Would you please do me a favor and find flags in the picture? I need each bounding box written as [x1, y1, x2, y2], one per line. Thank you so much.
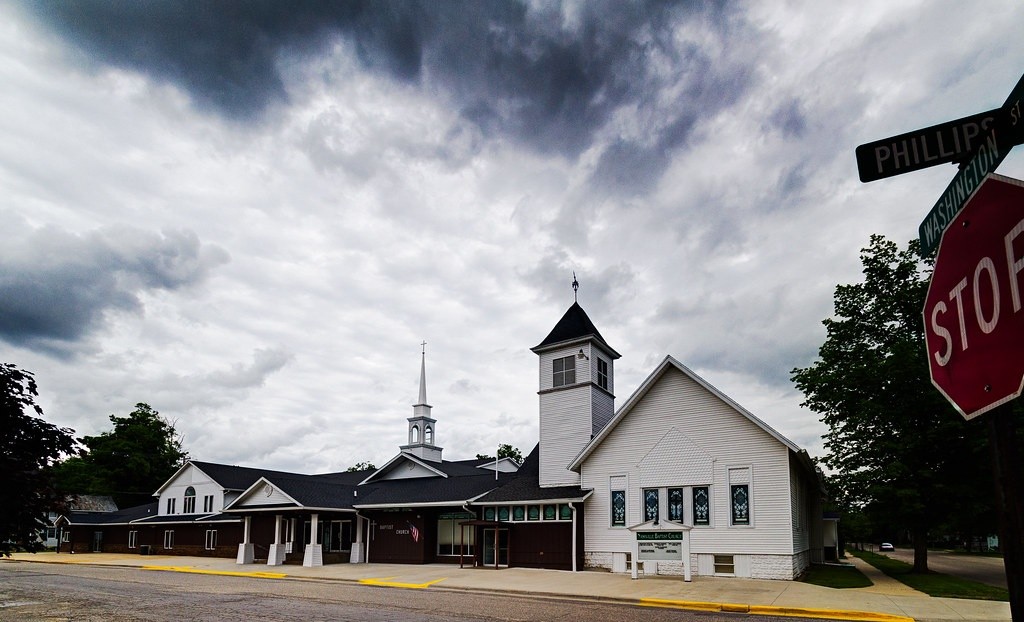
[409, 523, 419, 543]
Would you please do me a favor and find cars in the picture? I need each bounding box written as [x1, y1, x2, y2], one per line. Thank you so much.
[879, 543, 894, 551]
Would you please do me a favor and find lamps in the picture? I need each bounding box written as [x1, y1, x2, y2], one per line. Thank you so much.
[577, 349, 589, 360]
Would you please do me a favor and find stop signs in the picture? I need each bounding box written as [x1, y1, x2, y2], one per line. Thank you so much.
[921, 173, 1024, 421]
